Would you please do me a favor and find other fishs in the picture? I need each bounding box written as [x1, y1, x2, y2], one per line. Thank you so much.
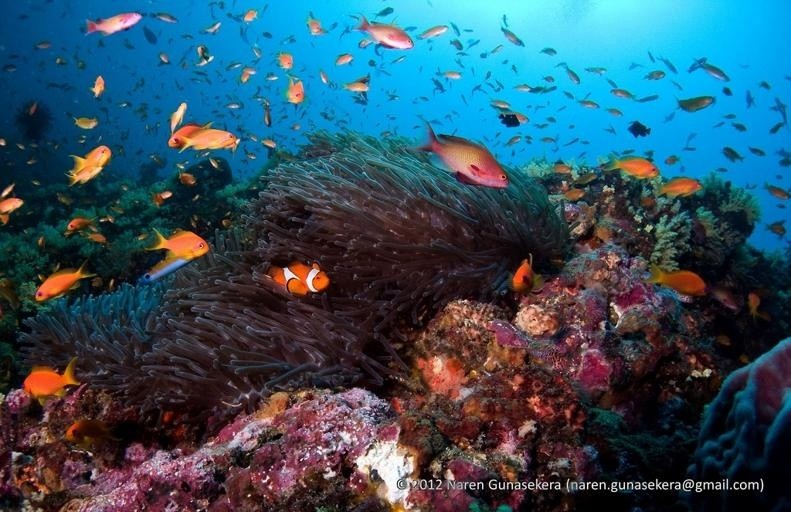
[0, 0, 790, 318]
